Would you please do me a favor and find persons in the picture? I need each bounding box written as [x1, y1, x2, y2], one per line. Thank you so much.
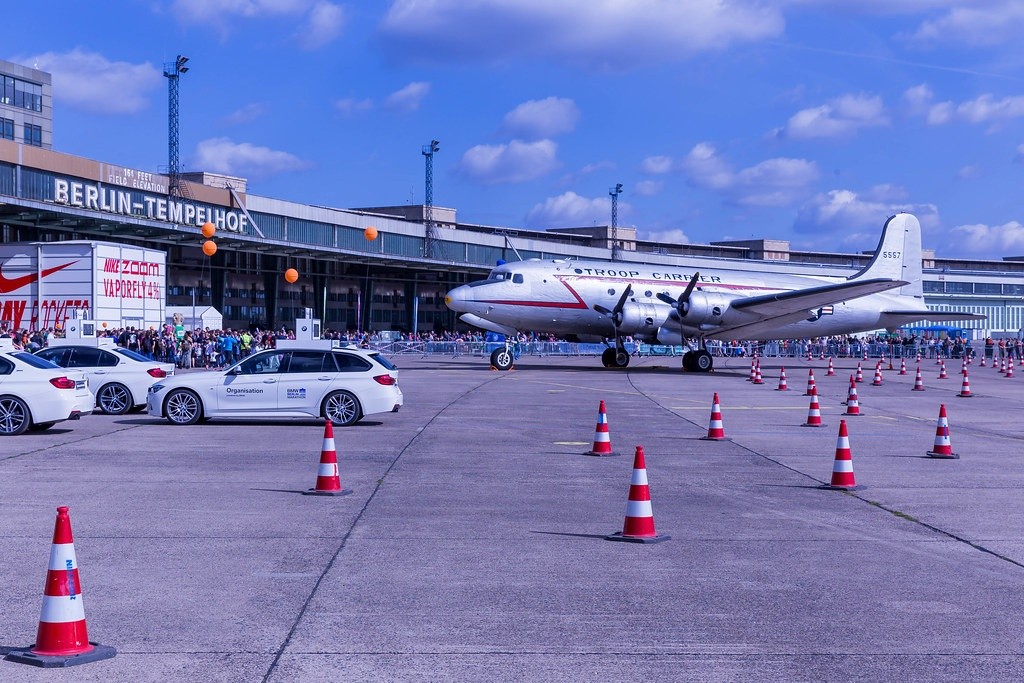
[665, 345, 676, 355]
[0, 325, 296, 369]
[320, 328, 381, 350]
[706, 335, 976, 359]
[400, 331, 568, 360]
[986, 337, 1024, 360]
[650, 345, 656, 354]
[631, 340, 642, 358]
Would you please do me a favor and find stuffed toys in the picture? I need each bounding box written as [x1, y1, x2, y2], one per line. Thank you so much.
[173, 313, 184, 350]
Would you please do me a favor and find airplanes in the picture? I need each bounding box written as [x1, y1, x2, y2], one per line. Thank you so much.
[444, 210, 999, 374]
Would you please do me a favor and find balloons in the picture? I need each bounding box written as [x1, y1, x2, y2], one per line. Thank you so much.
[364, 226, 378, 241]
[285, 269, 298, 283]
[102, 322, 107, 328]
[203, 241, 217, 257]
[201, 221, 215, 237]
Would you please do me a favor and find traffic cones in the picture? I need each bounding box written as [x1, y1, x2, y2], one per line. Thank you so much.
[605, 444, 672, 543]
[753, 359, 766, 384]
[840, 379, 865, 416]
[699, 391, 733, 441]
[583, 399, 621, 457]
[956, 369, 976, 397]
[800, 386, 827, 428]
[747, 360, 756, 381]
[774, 366, 791, 390]
[854, 349, 1024, 387]
[840, 373, 861, 405]
[807, 350, 814, 362]
[6, 506, 118, 669]
[819, 350, 826, 360]
[818, 418, 867, 490]
[753, 350, 758, 359]
[926, 403, 960, 459]
[302, 419, 354, 496]
[911, 366, 927, 391]
[824, 357, 838, 376]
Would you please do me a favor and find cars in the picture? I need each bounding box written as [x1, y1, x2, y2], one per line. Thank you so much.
[0, 345, 95, 437]
[145, 345, 403, 427]
[32, 341, 176, 416]
